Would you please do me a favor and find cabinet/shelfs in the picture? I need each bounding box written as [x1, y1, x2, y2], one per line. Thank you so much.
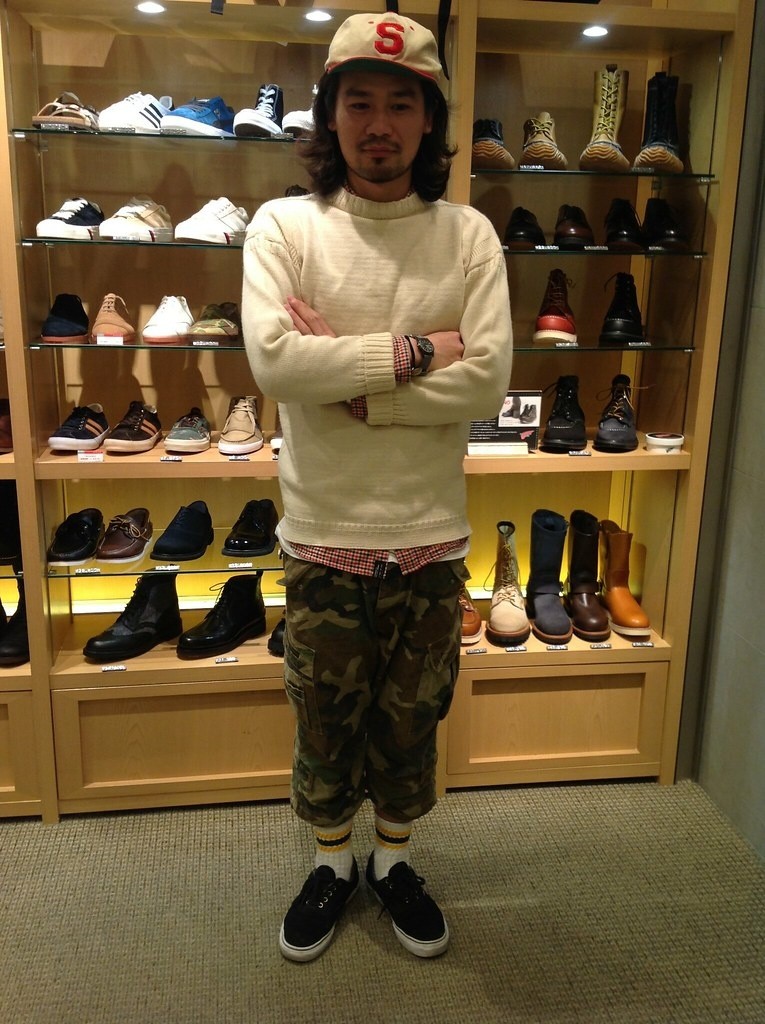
[0, 0, 757, 832]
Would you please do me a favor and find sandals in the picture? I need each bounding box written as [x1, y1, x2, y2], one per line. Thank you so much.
[31, 91, 99, 128]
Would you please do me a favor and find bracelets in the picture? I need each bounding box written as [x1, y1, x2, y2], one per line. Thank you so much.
[404, 336, 415, 370]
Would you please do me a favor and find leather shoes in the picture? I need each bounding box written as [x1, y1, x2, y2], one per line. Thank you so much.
[602, 198, 644, 249]
[553, 205, 594, 247]
[643, 196, 690, 248]
[46, 500, 279, 566]
[504, 206, 546, 249]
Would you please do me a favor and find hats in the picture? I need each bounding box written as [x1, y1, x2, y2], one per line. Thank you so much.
[324, 12, 442, 86]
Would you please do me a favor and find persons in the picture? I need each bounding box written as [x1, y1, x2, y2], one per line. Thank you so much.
[240, 12, 513, 963]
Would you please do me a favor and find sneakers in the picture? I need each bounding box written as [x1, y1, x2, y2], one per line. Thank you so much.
[471, 117, 515, 168]
[232, 82, 286, 136]
[364, 850, 449, 957]
[34, 195, 282, 454]
[97, 91, 174, 131]
[280, 853, 359, 962]
[161, 95, 236, 136]
[518, 112, 568, 168]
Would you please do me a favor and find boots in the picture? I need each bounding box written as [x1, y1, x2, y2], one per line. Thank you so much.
[533, 268, 579, 342]
[454, 509, 651, 643]
[594, 373, 649, 448]
[0, 561, 287, 663]
[599, 271, 643, 341]
[634, 72, 684, 173]
[578, 63, 630, 169]
[542, 375, 587, 447]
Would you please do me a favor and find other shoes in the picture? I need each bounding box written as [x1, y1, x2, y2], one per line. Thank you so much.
[281, 110, 317, 133]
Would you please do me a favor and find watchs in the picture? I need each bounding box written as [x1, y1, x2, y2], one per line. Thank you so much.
[409, 334, 434, 373]
